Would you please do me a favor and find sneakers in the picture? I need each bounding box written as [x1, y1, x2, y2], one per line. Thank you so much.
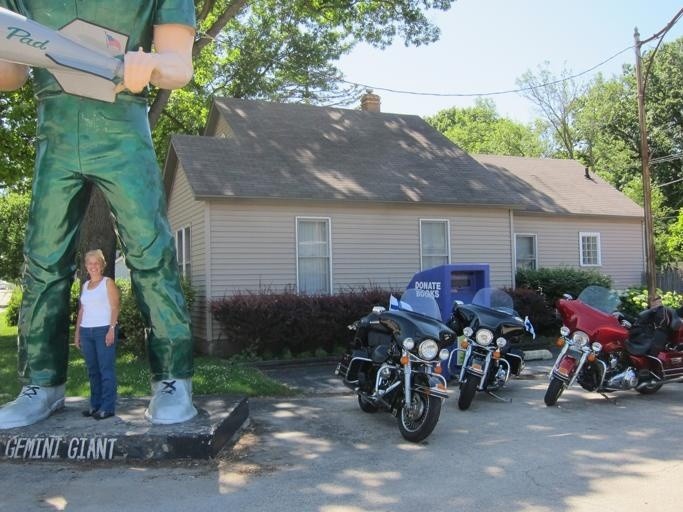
[82, 407, 97, 417]
[91, 410, 114, 419]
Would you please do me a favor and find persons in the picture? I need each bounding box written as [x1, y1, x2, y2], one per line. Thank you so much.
[72, 248, 121, 420]
[1, 0, 198, 430]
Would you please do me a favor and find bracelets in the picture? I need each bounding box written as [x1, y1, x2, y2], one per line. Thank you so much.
[110, 321, 117, 329]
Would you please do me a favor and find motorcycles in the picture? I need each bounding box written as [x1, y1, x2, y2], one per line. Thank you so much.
[541, 285, 683, 405]
[334, 289, 536, 442]
